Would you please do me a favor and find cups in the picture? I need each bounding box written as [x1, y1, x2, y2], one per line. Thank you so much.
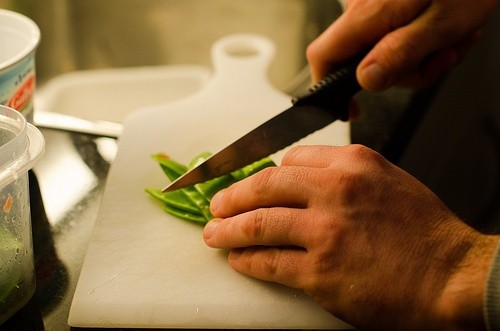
[0, 8, 41, 136]
[207, 36, 274, 90]
[0, 102, 46, 325]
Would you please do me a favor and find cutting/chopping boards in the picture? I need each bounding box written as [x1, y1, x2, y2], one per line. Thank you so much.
[68, 87, 353, 329]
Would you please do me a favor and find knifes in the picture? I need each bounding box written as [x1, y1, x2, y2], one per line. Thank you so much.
[162, 55, 367, 194]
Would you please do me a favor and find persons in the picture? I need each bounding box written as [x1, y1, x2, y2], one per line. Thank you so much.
[204, 0, 500, 331]
